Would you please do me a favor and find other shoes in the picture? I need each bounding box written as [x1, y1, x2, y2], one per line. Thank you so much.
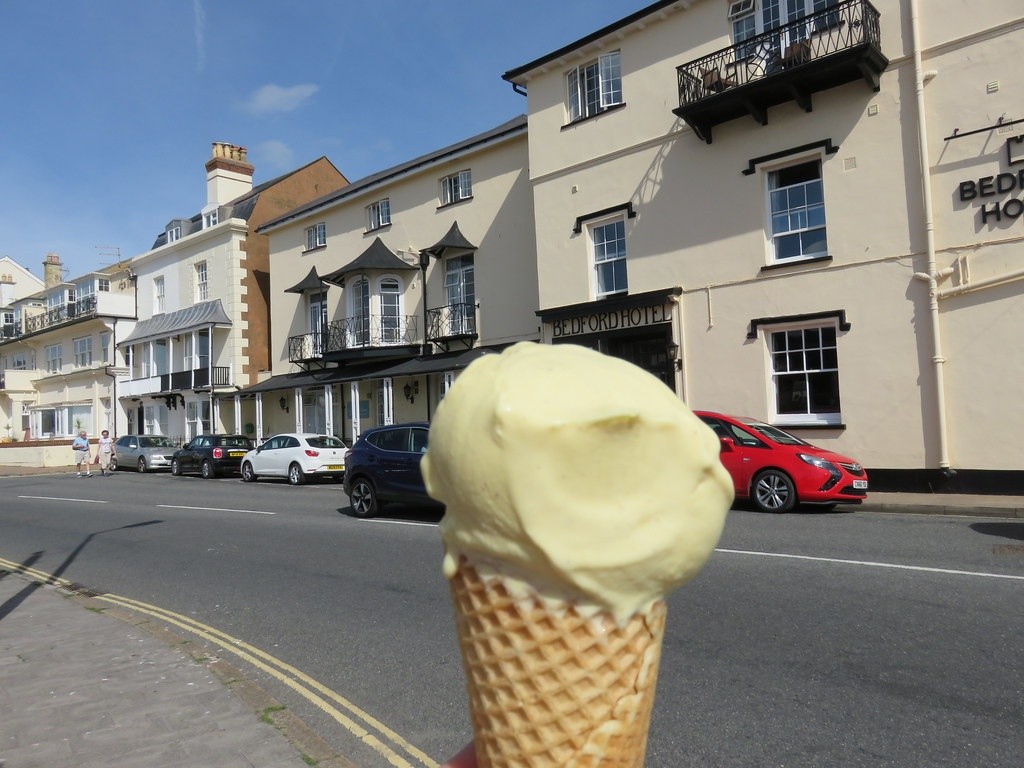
[77, 474, 82, 478]
[87, 474, 93, 477]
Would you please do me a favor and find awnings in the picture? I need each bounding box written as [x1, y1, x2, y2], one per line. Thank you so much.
[318, 235, 420, 288]
[418, 220, 479, 260]
[211, 338, 541, 399]
[283, 265, 330, 294]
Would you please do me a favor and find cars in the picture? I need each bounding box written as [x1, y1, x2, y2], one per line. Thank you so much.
[109, 434, 182, 473]
[692, 410, 869, 514]
[240, 433, 350, 486]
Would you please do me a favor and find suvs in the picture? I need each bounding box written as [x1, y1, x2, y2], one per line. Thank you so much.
[170, 434, 255, 479]
[341, 420, 447, 518]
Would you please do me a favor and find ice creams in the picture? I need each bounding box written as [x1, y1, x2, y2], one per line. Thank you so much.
[421, 342, 737, 768]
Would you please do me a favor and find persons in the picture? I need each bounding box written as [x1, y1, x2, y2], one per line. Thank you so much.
[95, 430, 117, 477]
[71, 431, 93, 478]
